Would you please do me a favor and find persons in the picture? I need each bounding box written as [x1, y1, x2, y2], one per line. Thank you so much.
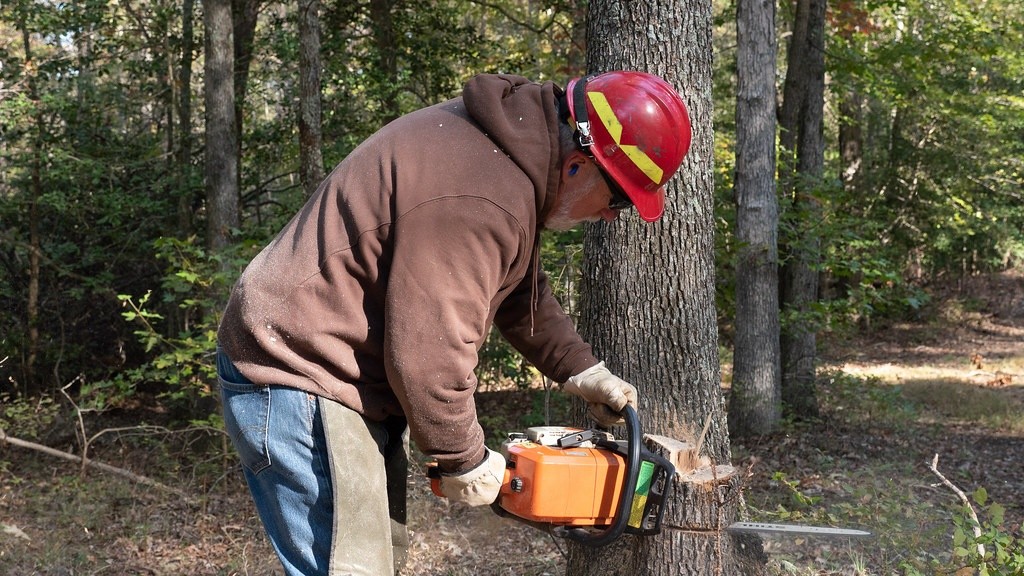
[218, 71, 692, 575]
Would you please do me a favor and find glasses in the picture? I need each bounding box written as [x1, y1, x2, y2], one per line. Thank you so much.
[575, 141, 635, 209]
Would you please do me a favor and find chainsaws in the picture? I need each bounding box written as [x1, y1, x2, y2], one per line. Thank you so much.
[426, 401, 873, 540]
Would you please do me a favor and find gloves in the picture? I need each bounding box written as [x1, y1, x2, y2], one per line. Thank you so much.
[563, 361, 636, 426]
[439, 445, 507, 506]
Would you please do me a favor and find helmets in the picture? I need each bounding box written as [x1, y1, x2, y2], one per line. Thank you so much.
[568, 71, 691, 224]
